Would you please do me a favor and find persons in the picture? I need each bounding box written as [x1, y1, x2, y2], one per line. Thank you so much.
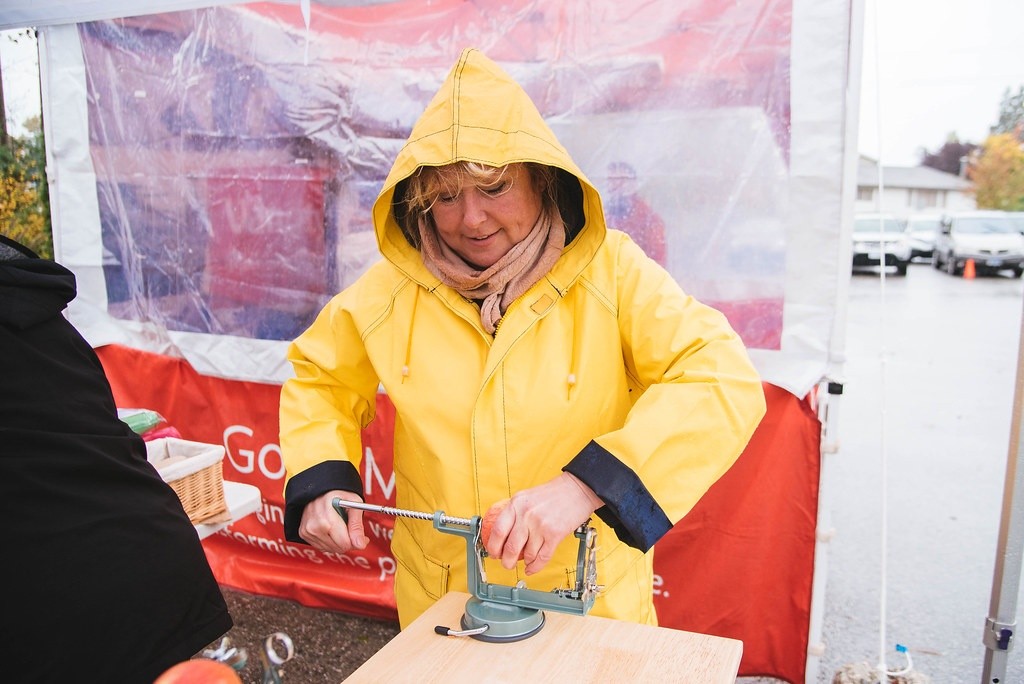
[280, 50, 770, 636]
[0, 79, 232, 684]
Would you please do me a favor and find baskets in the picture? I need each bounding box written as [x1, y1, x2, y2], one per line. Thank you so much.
[142, 436, 228, 526]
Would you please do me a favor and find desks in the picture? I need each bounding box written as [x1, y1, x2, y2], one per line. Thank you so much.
[341, 590, 743, 684]
[194, 481, 262, 542]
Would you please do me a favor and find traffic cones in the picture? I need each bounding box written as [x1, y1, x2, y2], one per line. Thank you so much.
[962, 257, 977, 280]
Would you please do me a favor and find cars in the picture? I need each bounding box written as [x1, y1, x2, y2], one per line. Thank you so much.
[931, 211, 1024, 276]
[849, 209, 912, 276]
[904, 208, 948, 265]
